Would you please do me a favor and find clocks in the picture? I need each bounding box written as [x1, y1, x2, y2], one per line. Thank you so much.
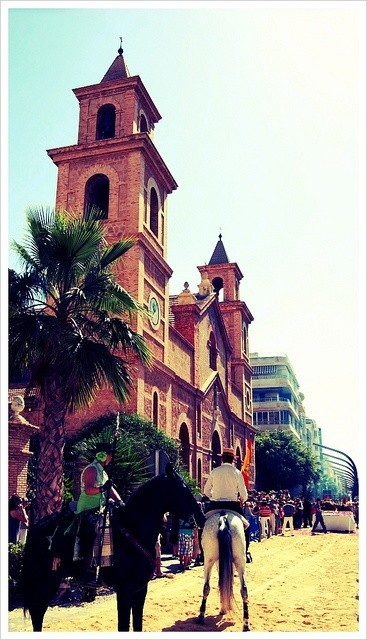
[147, 292, 161, 330]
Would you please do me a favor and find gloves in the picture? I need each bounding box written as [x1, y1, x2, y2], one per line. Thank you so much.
[99, 479, 113, 493]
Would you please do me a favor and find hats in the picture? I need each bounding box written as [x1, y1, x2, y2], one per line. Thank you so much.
[91, 442, 119, 454]
[260, 498, 267, 502]
[217, 448, 240, 458]
[12, 496, 21, 503]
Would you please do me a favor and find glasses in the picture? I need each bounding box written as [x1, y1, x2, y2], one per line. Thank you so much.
[17, 501, 24, 504]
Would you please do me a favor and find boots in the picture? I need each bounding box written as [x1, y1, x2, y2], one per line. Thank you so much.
[82, 551, 98, 572]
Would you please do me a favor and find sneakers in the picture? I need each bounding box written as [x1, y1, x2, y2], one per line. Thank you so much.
[246, 555, 251, 564]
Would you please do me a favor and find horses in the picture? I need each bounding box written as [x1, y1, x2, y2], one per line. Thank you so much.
[17, 457, 200, 631]
[198, 511, 250, 632]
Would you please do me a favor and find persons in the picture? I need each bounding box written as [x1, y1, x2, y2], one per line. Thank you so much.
[305, 497, 358, 533]
[248, 490, 303, 536]
[152, 533, 164, 580]
[164, 514, 202, 570]
[77, 442, 112, 566]
[9, 493, 28, 545]
[204, 448, 252, 564]
